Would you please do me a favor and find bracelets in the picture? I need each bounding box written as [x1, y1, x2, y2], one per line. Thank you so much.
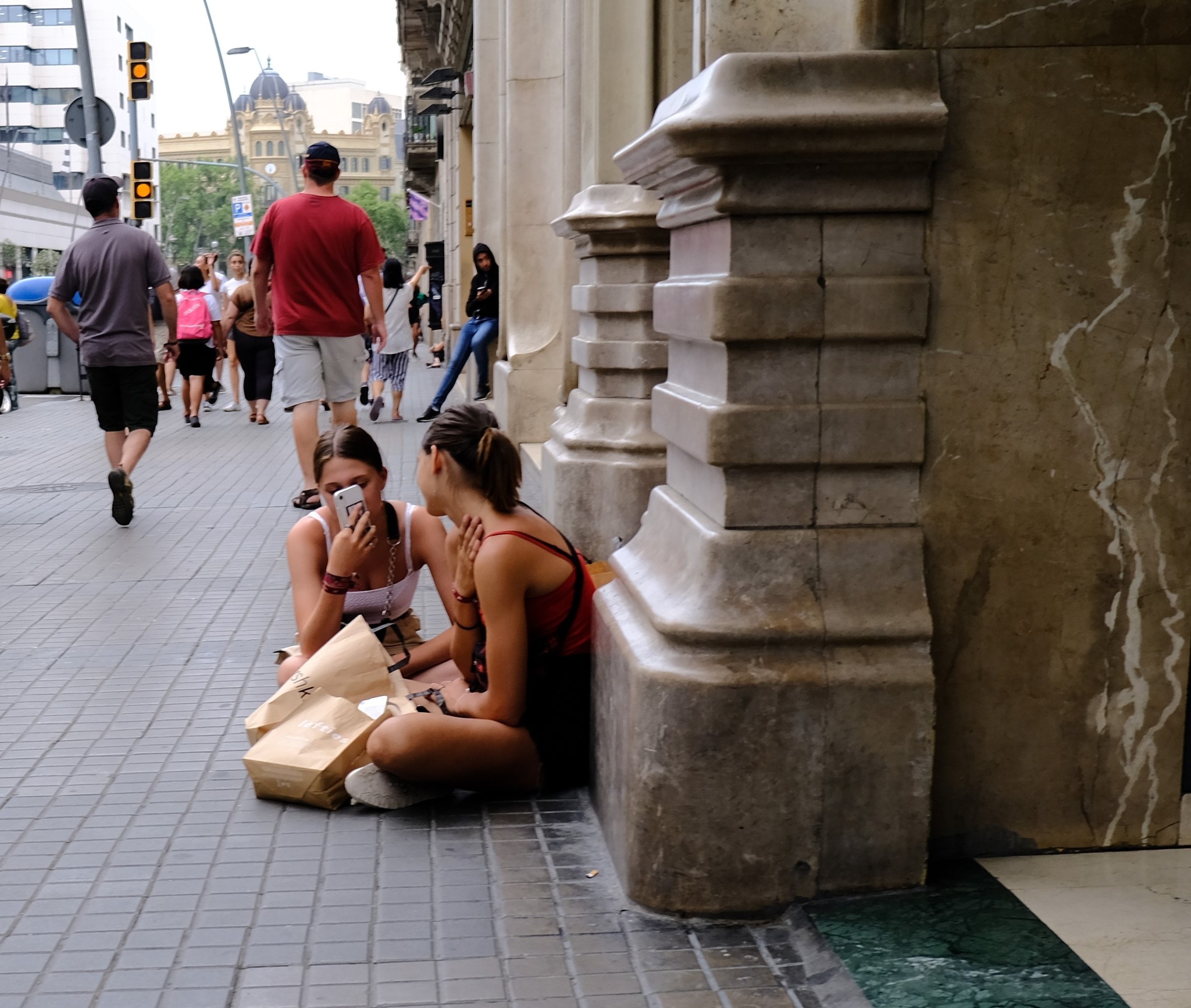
[454, 618, 478, 630]
[451, 586, 478, 603]
[0, 353, 12, 361]
[322, 572, 360, 594]
[164, 341, 178, 347]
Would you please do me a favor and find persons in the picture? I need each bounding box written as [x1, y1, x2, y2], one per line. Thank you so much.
[343, 403, 597, 811]
[272, 421, 465, 715]
[45, 178, 180, 526]
[417, 243, 499, 423]
[0, 278, 21, 415]
[147, 249, 444, 428]
[249, 142, 387, 510]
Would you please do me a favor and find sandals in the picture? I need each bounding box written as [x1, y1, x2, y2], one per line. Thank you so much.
[292, 488, 322, 510]
[249, 413, 257, 422]
[256, 414, 269, 425]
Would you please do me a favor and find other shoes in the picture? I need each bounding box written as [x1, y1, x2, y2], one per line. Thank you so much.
[426, 363, 432, 366]
[473, 383, 491, 401]
[392, 416, 403, 421]
[416, 406, 441, 422]
[321, 402, 330, 411]
[184, 413, 191, 423]
[203, 401, 211, 412]
[158, 400, 172, 411]
[223, 402, 241, 411]
[344, 763, 422, 810]
[108, 467, 134, 526]
[360, 382, 369, 405]
[427, 365, 441, 369]
[191, 416, 200, 428]
[274, 361, 284, 375]
[369, 396, 383, 421]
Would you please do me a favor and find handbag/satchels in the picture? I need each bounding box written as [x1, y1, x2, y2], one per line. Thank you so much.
[242, 686, 393, 811]
[243, 613, 451, 748]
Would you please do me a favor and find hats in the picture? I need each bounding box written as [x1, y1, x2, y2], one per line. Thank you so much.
[82, 174, 125, 201]
[305, 141, 340, 165]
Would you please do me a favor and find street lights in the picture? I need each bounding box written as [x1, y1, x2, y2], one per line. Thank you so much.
[226, 47, 300, 195]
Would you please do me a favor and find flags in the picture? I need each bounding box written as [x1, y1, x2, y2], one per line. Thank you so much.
[409, 190, 430, 220]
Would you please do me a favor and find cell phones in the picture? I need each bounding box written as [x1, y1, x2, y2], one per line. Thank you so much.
[334, 484, 373, 539]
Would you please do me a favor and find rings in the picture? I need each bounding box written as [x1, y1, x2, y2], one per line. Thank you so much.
[344, 524, 354, 530]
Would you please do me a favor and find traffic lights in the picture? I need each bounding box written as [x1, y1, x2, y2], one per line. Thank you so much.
[127, 41, 153, 100]
[132, 161, 156, 220]
